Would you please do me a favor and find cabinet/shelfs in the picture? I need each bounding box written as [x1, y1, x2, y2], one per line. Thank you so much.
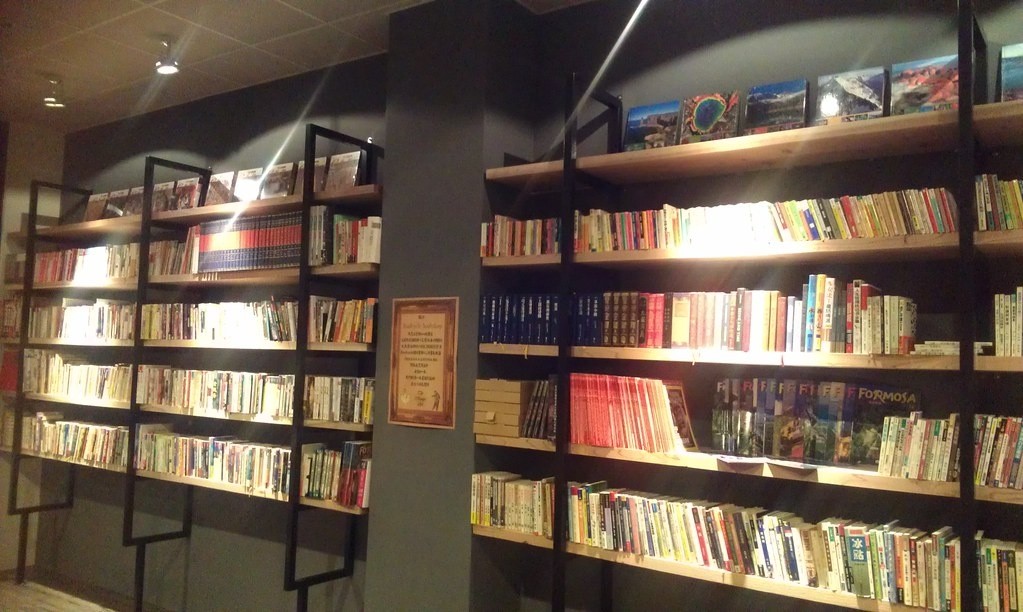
[470, 1, 1022, 612]
[8, 125, 385, 612]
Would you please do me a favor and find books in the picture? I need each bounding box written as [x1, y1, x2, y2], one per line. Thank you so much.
[5, 170, 1022, 611]
